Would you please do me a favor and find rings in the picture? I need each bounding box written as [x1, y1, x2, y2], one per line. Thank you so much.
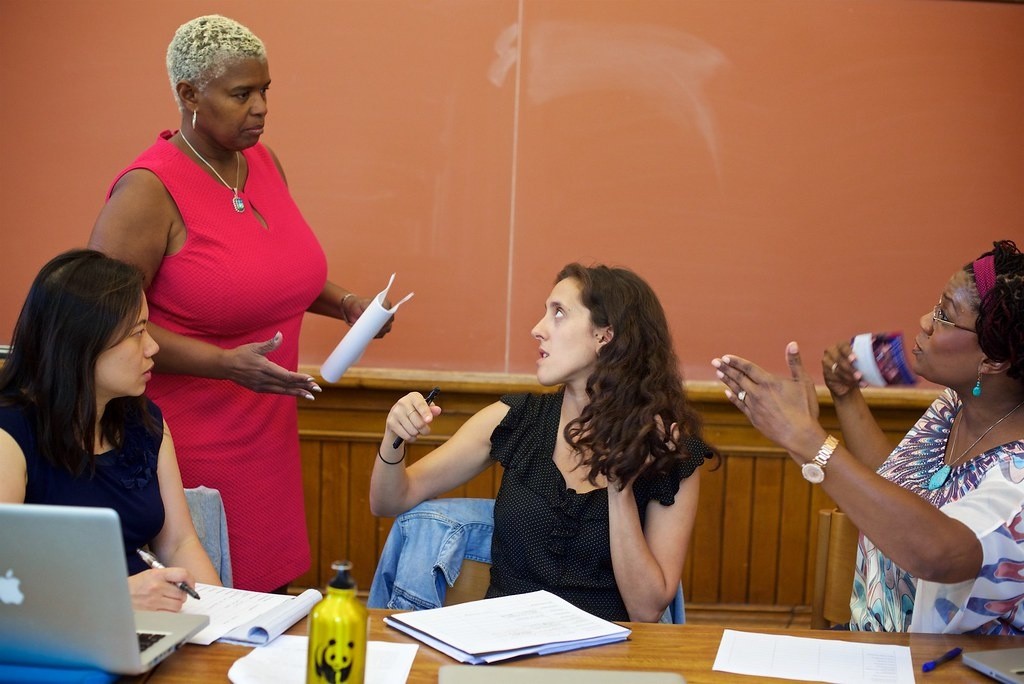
[738, 391, 746, 401]
[407, 409, 416, 417]
[831, 363, 838, 373]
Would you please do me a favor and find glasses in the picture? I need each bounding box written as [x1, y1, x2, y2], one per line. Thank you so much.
[929, 292, 981, 336]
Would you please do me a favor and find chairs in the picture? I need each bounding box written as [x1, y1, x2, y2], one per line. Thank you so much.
[811, 508, 860, 630]
[181, 484, 234, 589]
[366, 498, 686, 625]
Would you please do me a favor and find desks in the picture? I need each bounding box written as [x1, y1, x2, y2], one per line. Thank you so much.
[117, 604, 1024, 683]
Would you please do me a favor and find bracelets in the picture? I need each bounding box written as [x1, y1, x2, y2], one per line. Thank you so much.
[378, 447, 406, 465]
[341, 294, 355, 327]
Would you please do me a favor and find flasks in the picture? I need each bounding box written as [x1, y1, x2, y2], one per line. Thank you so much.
[305, 561, 366, 684]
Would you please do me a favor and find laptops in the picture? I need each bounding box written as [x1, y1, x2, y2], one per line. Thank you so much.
[0, 502, 211, 678]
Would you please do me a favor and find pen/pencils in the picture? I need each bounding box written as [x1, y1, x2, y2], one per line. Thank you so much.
[137, 548, 201, 600]
[392, 386, 441, 449]
[922, 647, 964, 673]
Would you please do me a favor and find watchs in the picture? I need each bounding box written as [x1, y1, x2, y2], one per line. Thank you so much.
[802, 434, 839, 484]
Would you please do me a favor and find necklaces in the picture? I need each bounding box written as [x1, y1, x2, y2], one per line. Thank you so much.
[928, 402, 1024, 491]
[179, 129, 245, 213]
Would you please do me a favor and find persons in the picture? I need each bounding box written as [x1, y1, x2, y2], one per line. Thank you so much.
[711, 240, 1024, 638]
[369, 261, 722, 623]
[0, 249, 222, 614]
[87, 14, 395, 596]
